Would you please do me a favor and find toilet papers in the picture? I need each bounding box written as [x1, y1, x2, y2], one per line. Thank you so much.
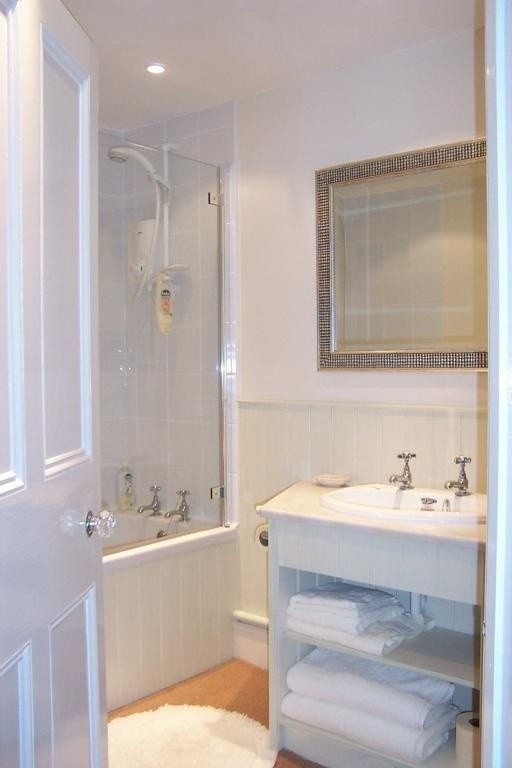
[455, 711, 481, 768]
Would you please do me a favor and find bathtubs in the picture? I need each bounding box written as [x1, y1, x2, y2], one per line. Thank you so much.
[103, 509, 237, 713]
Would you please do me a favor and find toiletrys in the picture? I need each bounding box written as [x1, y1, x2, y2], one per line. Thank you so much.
[117, 462, 136, 511]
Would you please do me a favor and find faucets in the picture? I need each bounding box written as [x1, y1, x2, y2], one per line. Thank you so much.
[138, 487, 161, 516]
[390, 452, 417, 490]
[164, 490, 191, 521]
[444, 456, 473, 496]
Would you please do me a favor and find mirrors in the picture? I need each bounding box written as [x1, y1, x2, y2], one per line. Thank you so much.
[315, 137, 486, 371]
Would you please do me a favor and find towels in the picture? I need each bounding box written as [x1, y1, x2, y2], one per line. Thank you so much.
[279, 690, 448, 762]
[283, 646, 462, 728]
[286, 581, 434, 656]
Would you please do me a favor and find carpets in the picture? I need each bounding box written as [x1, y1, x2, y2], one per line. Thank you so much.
[106, 700, 277, 767]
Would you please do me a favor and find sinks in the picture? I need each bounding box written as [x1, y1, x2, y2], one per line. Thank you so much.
[318, 483, 487, 525]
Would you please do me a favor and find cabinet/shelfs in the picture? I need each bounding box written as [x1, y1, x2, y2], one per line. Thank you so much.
[265, 515, 485, 767]
